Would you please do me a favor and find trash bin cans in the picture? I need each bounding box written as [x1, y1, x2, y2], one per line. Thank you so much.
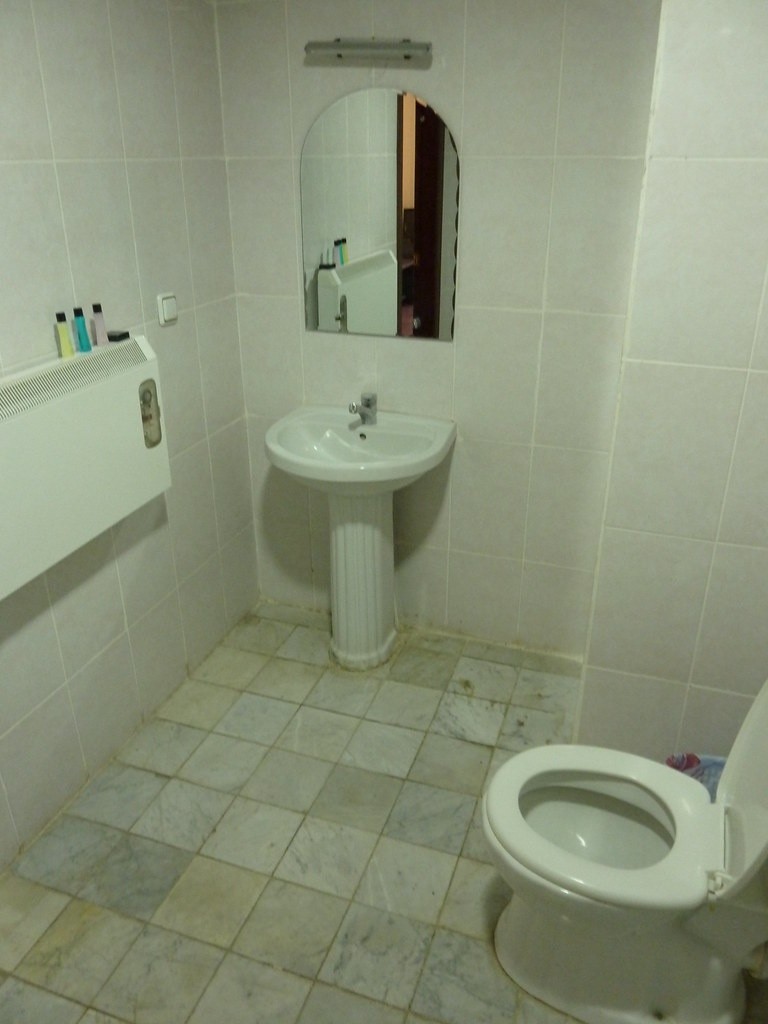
[665, 753, 728, 803]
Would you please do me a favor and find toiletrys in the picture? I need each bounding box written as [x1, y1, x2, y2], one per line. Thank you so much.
[92, 302, 111, 347]
[55, 311, 77, 359]
[333, 240, 342, 268]
[337, 239, 345, 266]
[73, 306, 94, 353]
[341, 237, 349, 265]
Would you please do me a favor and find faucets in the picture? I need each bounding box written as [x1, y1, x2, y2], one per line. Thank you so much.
[348, 391, 380, 425]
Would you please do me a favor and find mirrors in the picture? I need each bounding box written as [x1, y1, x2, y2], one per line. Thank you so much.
[300, 88, 459, 342]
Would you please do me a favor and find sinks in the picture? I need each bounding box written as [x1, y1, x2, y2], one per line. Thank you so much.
[264, 400, 458, 498]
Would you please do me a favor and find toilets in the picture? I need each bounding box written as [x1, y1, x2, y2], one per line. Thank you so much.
[475, 672, 768, 1024]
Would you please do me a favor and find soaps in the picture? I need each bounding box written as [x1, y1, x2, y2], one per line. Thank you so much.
[108, 328, 130, 343]
[318, 263, 338, 271]
